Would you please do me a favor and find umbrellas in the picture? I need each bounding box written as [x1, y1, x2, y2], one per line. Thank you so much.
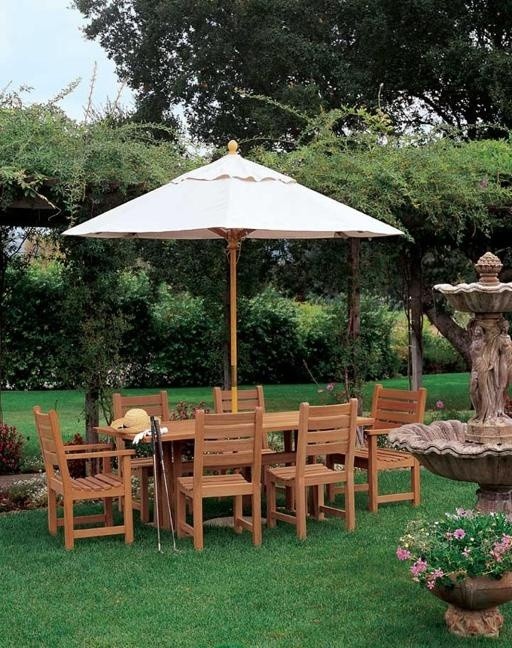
[59, 140, 406, 473]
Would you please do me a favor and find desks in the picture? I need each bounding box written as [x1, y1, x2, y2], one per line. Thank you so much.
[91, 410, 376, 532]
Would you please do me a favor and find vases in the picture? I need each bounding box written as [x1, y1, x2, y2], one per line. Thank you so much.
[422, 571, 511, 612]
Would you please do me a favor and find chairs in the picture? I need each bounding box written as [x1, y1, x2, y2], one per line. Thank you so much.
[266, 397, 358, 541]
[214, 383, 285, 509]
[176, 405, 264, 550]
[31, 404, 136, 550]
[113, 391, 193, 524]
[326, 383, 427, 513]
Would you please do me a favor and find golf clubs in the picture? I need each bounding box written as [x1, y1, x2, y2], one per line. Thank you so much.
[149, 415, 183, 555]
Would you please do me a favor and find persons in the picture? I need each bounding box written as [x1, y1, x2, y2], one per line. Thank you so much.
[467, 324, 488, 423]
[490, 318, 512, 419]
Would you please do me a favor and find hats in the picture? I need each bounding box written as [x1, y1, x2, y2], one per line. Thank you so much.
[111, 408, 160, 433]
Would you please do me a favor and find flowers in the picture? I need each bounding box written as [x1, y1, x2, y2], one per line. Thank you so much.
[395, 505, 512, 590]
